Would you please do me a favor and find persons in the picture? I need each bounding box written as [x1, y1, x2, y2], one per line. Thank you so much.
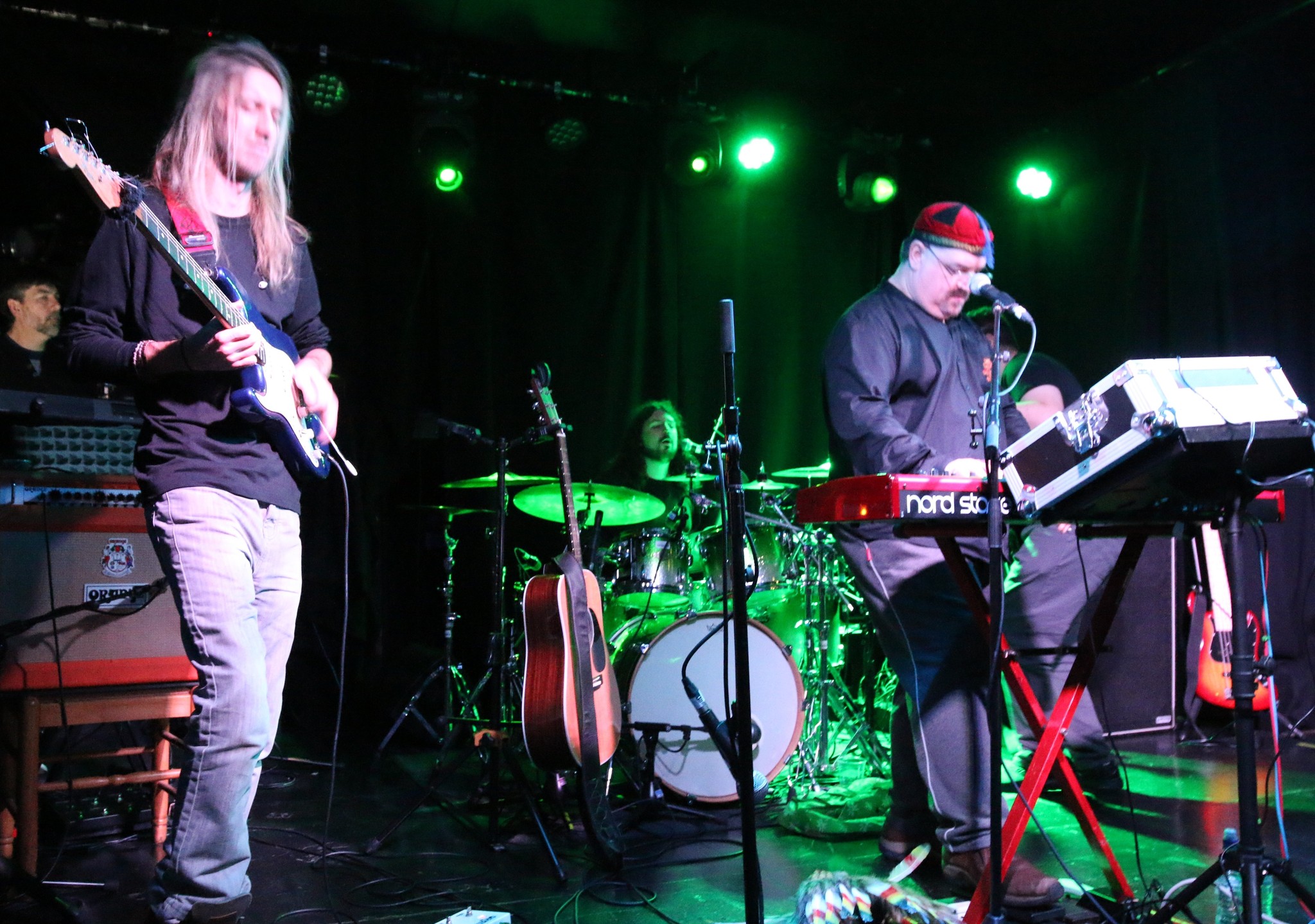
[67, 42, 341, 924]
[0, 265, 64, 395]
[821, 203, 1064, 906]
[602, 399, 728, 560]
[965, 306, 1130, 793]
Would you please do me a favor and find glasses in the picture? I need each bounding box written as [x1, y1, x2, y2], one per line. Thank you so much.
[928, 246, 993, 289]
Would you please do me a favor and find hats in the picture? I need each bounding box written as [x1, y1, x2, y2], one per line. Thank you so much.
[913, 201, 994, 257]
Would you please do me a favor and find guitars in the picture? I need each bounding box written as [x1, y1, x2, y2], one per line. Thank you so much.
[518, 359, 623, 774]
[39, 116, 334, 482]
[1185, 521, 1282, 712]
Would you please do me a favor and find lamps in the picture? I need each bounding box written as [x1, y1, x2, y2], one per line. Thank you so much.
[529, 100, 592, 160]
[836, 151, 900, 216]
[656, 122, 723, 182]
[431, 155, 472, 198]
[297, 58, 354, 116]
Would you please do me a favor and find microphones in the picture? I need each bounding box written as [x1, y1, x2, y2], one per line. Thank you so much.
[149, 577, 170, 595]
[751, 722, 761, 743]
[969, 273, 1033, 324]
[680, 438, 726, 459]
[682, 676, 769, 803]
[666, 496, 684, 525]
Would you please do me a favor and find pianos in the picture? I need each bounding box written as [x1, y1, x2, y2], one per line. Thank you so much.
[795, 468, 1151, 924]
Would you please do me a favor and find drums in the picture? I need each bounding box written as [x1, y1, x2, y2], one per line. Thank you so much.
[756, 581, 846, 676]
[696, 516, 790, 604]
[611, 527, 692, 613]
[607, 608, 806, 803]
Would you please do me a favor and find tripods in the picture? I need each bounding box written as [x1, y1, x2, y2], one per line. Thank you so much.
[1138, 471, 1314, 924]
[363, 420, 725, 887]
[753, 493, 894, 795]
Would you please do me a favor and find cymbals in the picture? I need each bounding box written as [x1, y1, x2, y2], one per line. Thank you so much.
[512, 480, 667, 528]
[439, 471, 563, 490]
[770, 467, 829, 479]
[657, 473, 717, 483]
[742, 480, 800, 491]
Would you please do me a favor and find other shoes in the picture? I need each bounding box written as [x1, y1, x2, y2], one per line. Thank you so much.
[146, 864, 242, 924]
[874, 829, 915, 861]
[1077, 764, 1124, 811]
[942, 846, 1064, 907]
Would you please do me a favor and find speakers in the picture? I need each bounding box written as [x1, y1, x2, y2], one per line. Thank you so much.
[0, 471, 199, 691]
[1089, 529, 1191, 738]
